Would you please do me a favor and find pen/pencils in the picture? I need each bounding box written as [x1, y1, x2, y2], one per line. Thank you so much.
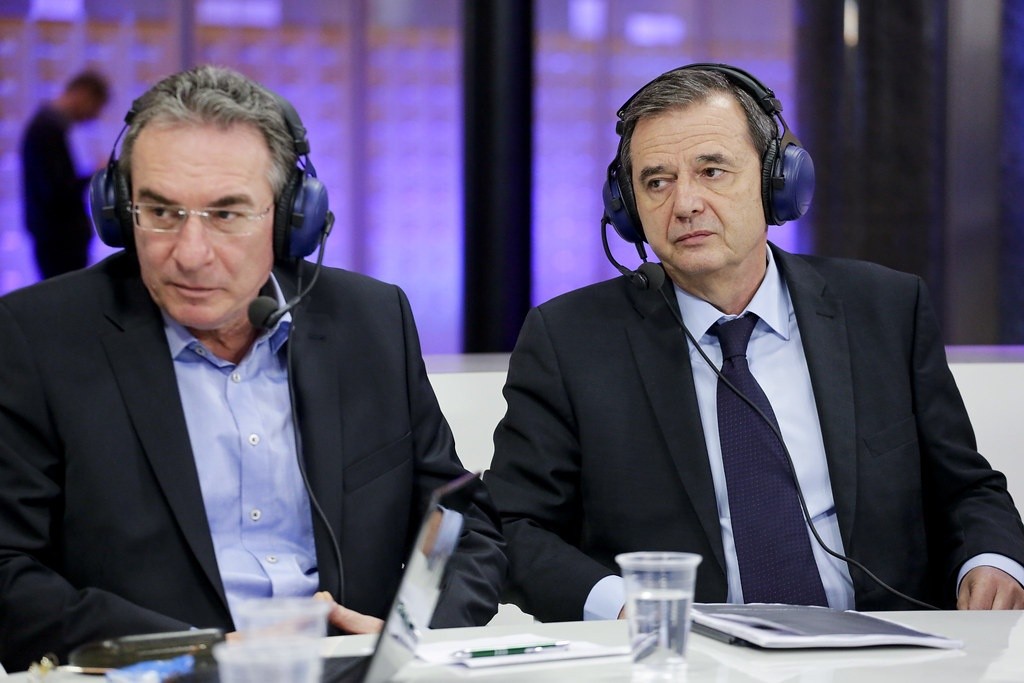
[454, 642, 574, 658]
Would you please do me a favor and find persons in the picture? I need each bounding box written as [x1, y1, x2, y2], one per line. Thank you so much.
[0, 66, 510, 674]
[484, 68, 1024, 624]
[21, 72, 108, 279]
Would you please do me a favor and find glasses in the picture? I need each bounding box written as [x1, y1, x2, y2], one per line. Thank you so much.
[125, 197, 275, 237]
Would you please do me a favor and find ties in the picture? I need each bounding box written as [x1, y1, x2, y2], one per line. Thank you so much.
[706, 313, 828, 607]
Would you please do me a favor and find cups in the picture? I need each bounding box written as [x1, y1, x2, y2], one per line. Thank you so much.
[615, 552, 702, 683]
[237, 596, 332, 683]
[212, 641, 321, 682]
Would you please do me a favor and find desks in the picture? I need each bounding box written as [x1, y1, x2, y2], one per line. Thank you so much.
[1, 607, 1022, 683]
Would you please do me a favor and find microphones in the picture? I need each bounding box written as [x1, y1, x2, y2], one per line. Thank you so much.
[599, 216, 664, 292]
[246, 210, 336, 328]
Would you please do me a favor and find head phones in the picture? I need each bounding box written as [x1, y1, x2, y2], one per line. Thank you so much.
[598, 63, 814, 246]
[91, 80, 328, 273]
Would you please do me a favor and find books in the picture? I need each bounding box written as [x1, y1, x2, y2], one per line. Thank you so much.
[691, 604, 963, 648]
